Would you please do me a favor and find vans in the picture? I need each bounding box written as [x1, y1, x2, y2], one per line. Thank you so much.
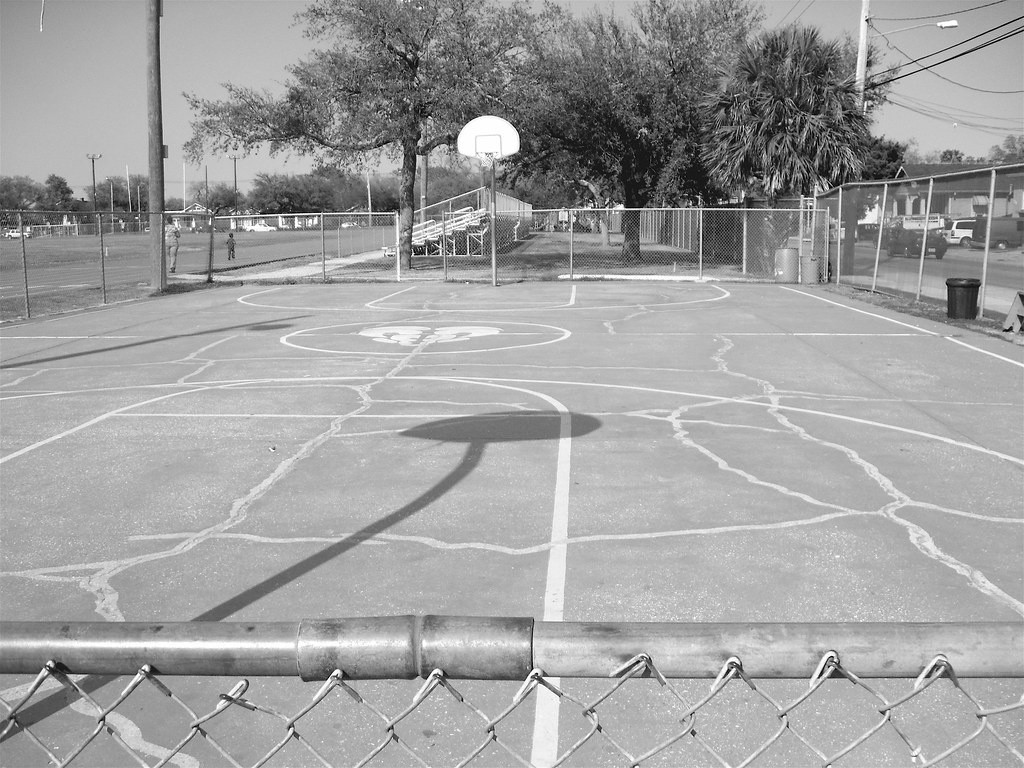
[968, 218, 1024, 249]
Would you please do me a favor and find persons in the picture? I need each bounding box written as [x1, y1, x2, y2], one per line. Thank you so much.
[226, 233, 236, 260]
[164, 216, 181, 273]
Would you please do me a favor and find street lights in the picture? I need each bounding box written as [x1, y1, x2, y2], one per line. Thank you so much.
[228, 155, 240, 232]
[86, 154, 102, 236]
[842, 20, 958, 274]
[106, 177, 114, 233]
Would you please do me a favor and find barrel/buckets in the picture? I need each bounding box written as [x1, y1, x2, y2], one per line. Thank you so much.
[775, 248, 798, 284]
[800, 256, 820, 283]
[945, 278, 981, 318]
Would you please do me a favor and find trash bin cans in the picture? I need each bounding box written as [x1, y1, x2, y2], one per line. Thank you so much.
[774, 247, 798, 285]
[800, 255, 820, 285]
[945, 277, 981, 320]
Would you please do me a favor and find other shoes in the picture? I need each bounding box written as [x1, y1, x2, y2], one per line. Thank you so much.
[170, 269, 175, 273]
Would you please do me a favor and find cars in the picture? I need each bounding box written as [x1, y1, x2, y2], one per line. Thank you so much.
[823, 221, 857, 245]
[190, 224, 226, 233]
[1, 229, 32, 239]
[873, 226, 895, 250]
[341, 222, 361, 229]
[887, 229, 948, 259]
[941, 219, 976, 246]
[246, 223, 277, 232]
